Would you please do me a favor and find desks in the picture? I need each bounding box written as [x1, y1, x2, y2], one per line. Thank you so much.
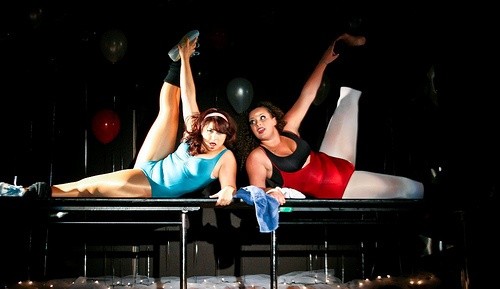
[40, 197, 430, 289]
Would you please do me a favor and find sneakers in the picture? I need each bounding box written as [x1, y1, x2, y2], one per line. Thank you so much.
[0, 176, 26, 198]
[168, 30, 200, 62]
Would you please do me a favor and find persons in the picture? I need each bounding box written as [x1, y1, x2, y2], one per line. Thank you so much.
[0, 31, 238, 206]
[237, 32, 424, 205]
